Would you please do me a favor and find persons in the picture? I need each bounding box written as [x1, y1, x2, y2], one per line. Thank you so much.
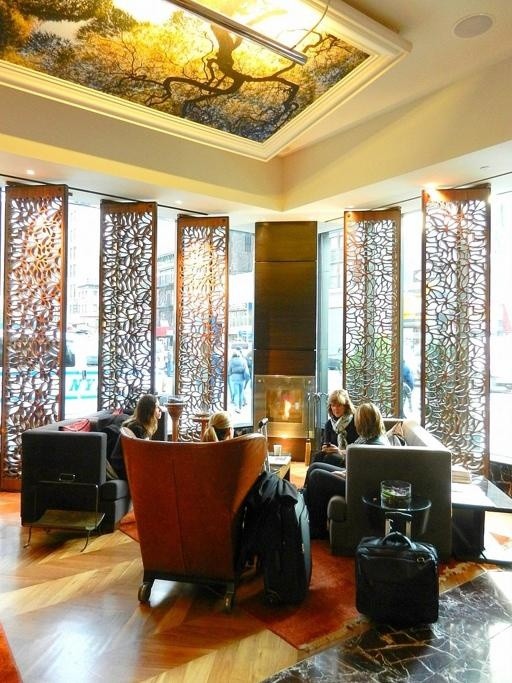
[301, 400, 394, 539]
[296, 387, 359, 494]
[225, 330, 416, 415]
[200, 410, 236, 442]
[110, 392, 163, 485]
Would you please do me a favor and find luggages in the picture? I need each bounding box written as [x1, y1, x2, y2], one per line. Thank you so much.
[354, 511, 440, 624]
[257, 417, 312, 609]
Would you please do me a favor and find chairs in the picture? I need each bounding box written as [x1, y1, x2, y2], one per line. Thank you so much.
[24, 471, 108, 554]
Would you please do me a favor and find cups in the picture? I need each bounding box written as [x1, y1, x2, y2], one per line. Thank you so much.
[273, 444, 281, 455]
[322, 441, 330, 453]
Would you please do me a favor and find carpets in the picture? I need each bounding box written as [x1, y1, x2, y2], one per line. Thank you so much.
[118, 477, 509, 652]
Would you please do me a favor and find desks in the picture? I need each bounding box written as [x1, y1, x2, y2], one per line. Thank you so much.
[161, 399, 210, 443]
[451, 470, 512, 569]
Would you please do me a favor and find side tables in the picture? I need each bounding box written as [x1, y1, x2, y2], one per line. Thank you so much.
[359, 479, 433, 544]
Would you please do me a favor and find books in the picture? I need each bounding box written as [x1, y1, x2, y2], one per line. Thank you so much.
[451, 465, 473, 484]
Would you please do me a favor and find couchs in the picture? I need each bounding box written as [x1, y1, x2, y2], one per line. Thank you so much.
[20, 406, 168, 535]
[116, 431, 269, 612]
[326, 417, 452, 561]
